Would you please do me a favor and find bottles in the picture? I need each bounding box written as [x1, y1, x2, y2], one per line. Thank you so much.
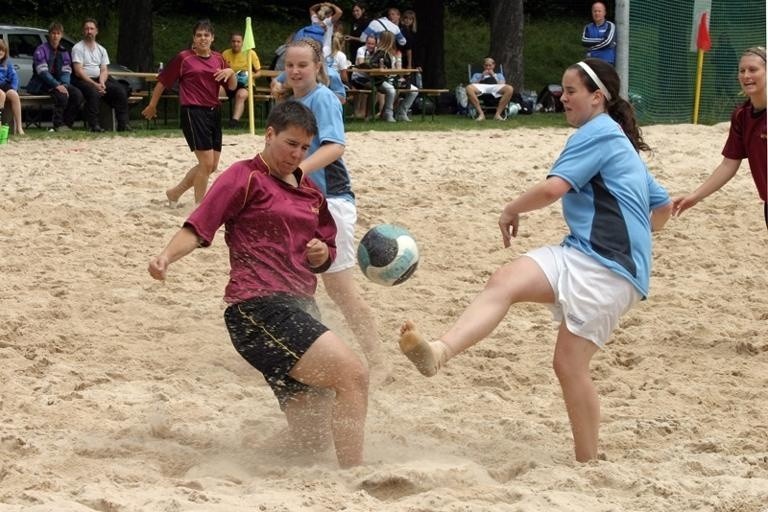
[378, 58, 385, 70]
[158, 63, 164, 73]
[416, 74, 422, 89]
[364, 52, 370, 64]
[529, 91, 538, 109]
[395, 51, 402, 70]
[0, 122, 10, 144]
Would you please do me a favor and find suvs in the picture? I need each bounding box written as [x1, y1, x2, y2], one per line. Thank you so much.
[269, 47, 439, 117]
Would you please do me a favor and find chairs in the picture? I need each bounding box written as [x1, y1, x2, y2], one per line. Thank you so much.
[465, 62, 509, 124]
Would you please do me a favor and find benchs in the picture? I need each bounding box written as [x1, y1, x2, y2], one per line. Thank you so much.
[13, 65, 451, 131]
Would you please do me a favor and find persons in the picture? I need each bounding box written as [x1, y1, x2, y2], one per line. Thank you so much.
[0, 38, 26, 135]
[149, 101, 370, 471]
[270, 0, 422, 124]
[219, 32, 262, 128]
[398, 58, 673, 463]
[581, 2, 617, 68]
[283, 40, 393, 386]
[140, 20, 238, 204]
[669, 44, 766, 229]
[26, 21, 85, 133]
[465, 56, 514, 122]
[71, 17, 133, 132]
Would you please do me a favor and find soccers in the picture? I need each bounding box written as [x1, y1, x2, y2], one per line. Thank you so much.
[357, 224, 420, 286]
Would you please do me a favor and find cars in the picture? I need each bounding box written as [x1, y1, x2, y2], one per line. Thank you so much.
[0, 25, 143, 113]
[538, 80, 645, 113]
